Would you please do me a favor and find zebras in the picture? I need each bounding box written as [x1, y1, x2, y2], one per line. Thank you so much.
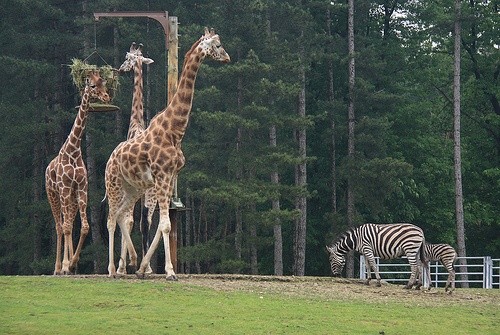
[325, 223, 458, 294]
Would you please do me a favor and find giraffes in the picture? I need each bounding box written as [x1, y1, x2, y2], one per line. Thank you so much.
[44, 25, 231, 281]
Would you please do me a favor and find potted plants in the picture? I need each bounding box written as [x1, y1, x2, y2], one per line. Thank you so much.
[68, 57, 121, 113]
